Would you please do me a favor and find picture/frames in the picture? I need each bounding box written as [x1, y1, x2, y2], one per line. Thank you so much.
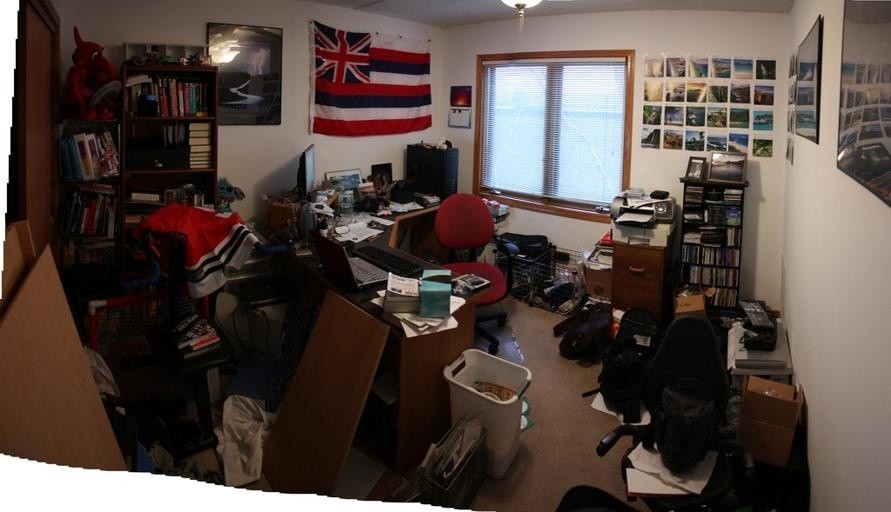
[204, 21, 287, 127]
[708, 150, 749, 184]
[794, 12, 824, 143]
[685, 156, 707, 178]
[325, 168, 363, 184]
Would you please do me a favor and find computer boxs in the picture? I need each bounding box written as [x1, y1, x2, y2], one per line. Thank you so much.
[407, 143, 458, 202]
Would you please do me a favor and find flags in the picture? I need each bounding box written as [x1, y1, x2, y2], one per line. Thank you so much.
[309, 17, 432, 137]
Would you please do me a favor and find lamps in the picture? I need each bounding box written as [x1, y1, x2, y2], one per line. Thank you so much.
[501, 0, 546, 32]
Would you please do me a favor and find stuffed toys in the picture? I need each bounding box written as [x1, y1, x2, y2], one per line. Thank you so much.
[70, 26, 120, 120]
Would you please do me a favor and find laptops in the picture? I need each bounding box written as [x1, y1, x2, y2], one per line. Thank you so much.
[308, 228, 391, 289]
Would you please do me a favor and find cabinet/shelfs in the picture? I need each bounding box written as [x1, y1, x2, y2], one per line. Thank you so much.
[120, 64, 220, 233]
[49, 118, 122, 300]
[679, 178, 749, 312]
[218, 253, 287, 308]
[612, 222, 677, 324]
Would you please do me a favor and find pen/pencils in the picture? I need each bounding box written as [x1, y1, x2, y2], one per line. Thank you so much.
[366, 234, 384, 243]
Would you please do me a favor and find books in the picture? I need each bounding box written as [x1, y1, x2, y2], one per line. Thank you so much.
[680, 185, 743, 307]
[59, 74, 213, 320]
[727, 322, 794, 375]
[383, 271, 420, 315]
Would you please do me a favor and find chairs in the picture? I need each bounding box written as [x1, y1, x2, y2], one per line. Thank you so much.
[589, 314, 737, 511]
[433, 192, 510, 354]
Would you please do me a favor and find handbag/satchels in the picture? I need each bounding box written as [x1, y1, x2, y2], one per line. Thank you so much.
[553, 302, 612, 363]
[582, 350, 644, 423]
[643, 385, 715, 475]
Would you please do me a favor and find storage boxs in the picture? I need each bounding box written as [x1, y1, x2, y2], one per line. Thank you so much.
[738, 372, 807, 468]
[583, 249, 614, 297]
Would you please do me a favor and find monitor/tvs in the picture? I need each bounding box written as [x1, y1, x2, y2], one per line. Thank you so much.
[297, 144, 316, 201]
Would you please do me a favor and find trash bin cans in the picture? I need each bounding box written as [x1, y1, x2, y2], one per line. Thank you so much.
[442, 347, 533, 480]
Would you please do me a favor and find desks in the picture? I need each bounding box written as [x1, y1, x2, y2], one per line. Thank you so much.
[268, 185, 492, 468]
